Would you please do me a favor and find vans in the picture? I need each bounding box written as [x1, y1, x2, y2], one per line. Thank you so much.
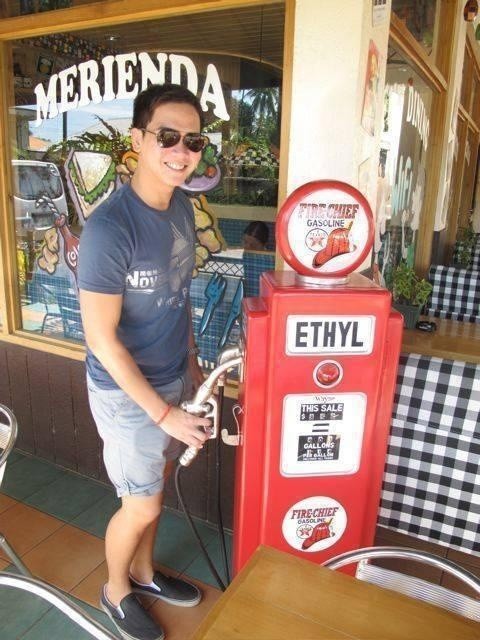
[11, 159, 69, 245]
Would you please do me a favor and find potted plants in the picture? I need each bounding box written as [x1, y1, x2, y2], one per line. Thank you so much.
[387, 255, 433, 330]
[451, 207, 476, 269]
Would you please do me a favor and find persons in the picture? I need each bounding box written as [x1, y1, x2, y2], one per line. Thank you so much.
[75, 82, 214, 639]
[239, 222, 269, 253]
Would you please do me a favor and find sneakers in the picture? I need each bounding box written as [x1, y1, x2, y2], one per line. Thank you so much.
[99, 583, 166, 640]
[127, 569, 202, 608]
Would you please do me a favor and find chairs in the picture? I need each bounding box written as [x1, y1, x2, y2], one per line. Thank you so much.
[375, 353, 480, 562]
[0, 402, 38, 582]
[51, 292, 85, 344]
[424, 263, 480, 322]
[318, 546, 480, 627]
[451, 240, 480, 272]
[32, 273, 72, 333]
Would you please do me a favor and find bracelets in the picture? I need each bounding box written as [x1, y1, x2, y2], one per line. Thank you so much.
[186, 344, 202, 356]
[152, 402, 173, 425]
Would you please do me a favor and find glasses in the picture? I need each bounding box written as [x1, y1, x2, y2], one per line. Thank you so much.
[140, 127, 205, 153]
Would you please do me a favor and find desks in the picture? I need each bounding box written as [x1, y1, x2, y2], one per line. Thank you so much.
[186, 543, 480, 640]
[399, 315, 480, 364]
